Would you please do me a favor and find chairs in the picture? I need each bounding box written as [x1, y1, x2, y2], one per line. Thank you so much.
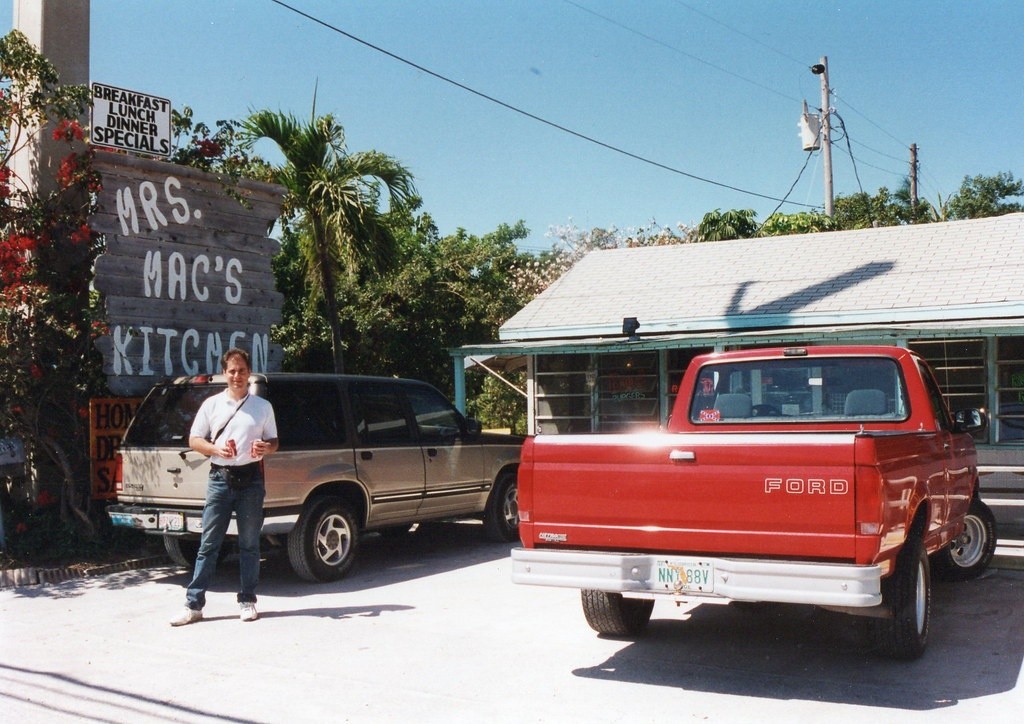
[844, 388, 888, 416]
[713, 393, 752, 418]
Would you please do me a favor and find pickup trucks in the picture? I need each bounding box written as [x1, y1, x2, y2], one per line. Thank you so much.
[511, 346, 998, 660]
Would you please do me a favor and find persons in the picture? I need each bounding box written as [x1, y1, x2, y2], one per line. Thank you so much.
[171, 347, 280, 627]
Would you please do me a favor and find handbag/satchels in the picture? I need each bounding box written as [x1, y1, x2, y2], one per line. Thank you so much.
[217, 466, 255, 487]
[204, 432, 214, 458]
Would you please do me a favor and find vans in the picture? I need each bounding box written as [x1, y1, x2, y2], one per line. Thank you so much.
[106, 372, 532, 581]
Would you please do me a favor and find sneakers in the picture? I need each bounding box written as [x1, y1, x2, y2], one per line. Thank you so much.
[170, 607, 203, 626]
[240, 602, 257, 621]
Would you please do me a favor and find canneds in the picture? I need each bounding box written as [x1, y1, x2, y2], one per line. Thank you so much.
[225, 439, 237, 457]
[251, 438, 262, 458]
